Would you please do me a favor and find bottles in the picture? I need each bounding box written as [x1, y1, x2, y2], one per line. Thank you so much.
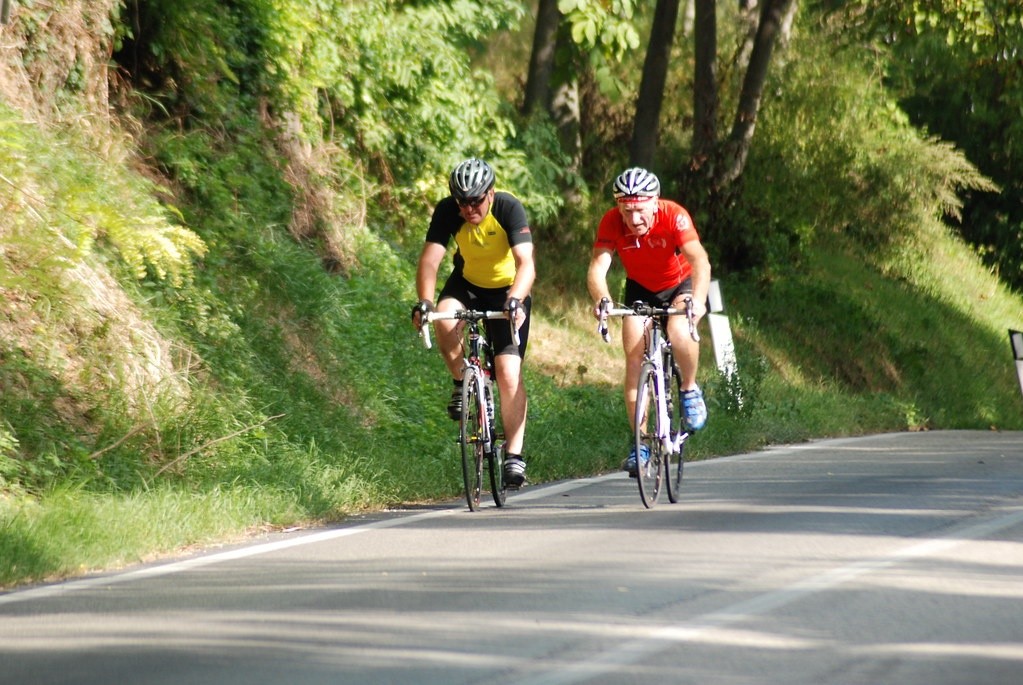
[483, 369, 495, 429]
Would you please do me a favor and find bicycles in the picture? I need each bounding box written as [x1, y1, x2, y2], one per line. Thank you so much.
[419, 308, 521, 513]
[597, 297, 701, 509]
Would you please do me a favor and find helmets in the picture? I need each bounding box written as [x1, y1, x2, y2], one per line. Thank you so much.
[447, 158, 496, 200]
[611, 167, 661, 199]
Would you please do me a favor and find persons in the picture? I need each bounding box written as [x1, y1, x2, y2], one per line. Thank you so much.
[411, 157, 536, 484]
[587, 165, 711, 471]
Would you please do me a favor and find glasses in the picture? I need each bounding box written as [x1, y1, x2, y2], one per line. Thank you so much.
[451, 190, 488, 208]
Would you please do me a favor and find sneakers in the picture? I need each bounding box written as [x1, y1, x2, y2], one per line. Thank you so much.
[447, 386, 473, 420]
[503, 450, 527, 486]
[623, 442, 652, 471]
[678, 380, 707, 432]
